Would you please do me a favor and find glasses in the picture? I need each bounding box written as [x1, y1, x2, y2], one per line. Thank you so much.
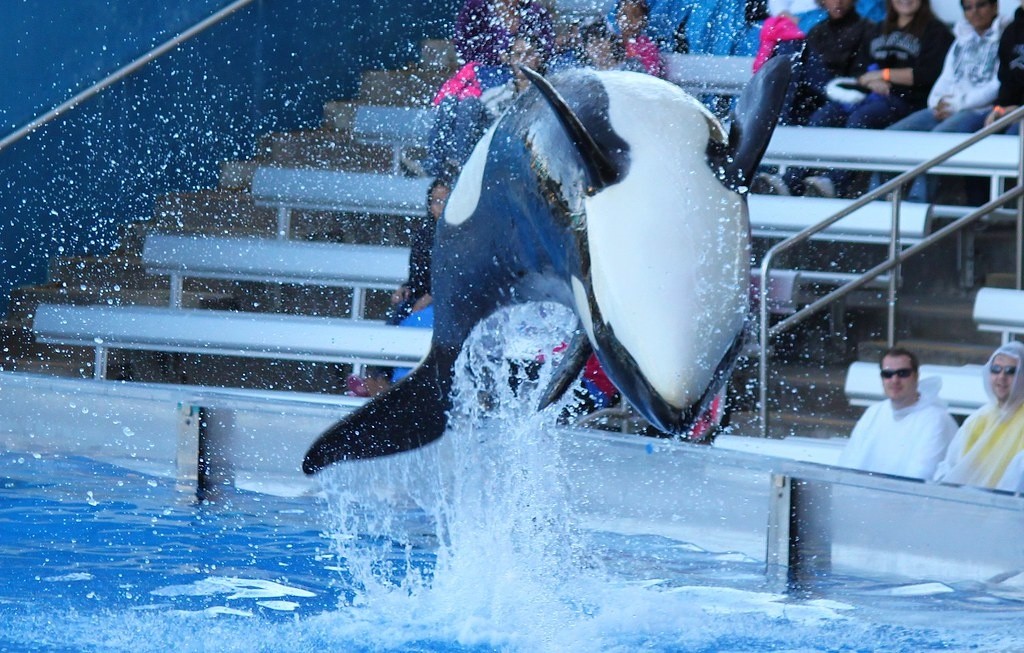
[990, 364, 1016, 374]
[881, 368, 913, 378]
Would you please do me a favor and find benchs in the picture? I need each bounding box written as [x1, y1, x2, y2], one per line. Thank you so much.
[33, 0, 1024, 481]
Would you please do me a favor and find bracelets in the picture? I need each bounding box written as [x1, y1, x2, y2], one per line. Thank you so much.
[882, 67, 890, 82]
[994, 105, 1006, 115]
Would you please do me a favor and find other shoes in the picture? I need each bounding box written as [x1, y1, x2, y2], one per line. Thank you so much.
[399, 156, 430, 177]
[348, 375, 392, 397]
[329, 363, 347, 387]
[802, 177, 840, 198]
[444, 160, 461, 176]
[749, 172, 790, 195]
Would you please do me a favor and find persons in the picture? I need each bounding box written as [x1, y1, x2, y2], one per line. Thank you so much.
[756, 0, 954, 200]
[936, 340, 1024, 495]
[841, 349, 958, 478]
[747, 1, 883, 193]
[347, 179, 451, 397]
[937, 0, 1024, 209]
[864, 0, 1014, 204]
[426, 1, 668, 183]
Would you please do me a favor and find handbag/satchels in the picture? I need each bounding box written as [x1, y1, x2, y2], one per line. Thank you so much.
[849, 55, 926, 107]
[434, 62, 484, 104]
[753, 15, 803, 72]
